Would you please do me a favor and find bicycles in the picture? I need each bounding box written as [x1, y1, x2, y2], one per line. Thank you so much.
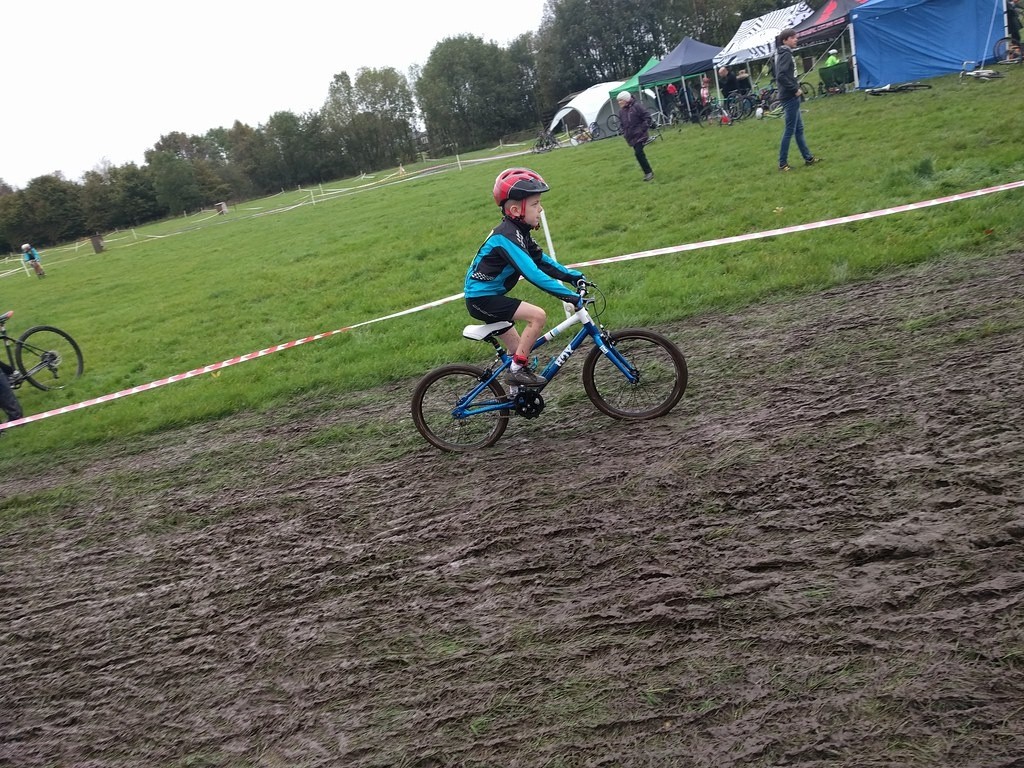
[529, 77, 846, 154]
[410, 279, 690, 456]
[865, 81, 932, 97]
[958, 60, 1005, 85]
[0, 310, 83, 394]
[994, 23, 1024, 65]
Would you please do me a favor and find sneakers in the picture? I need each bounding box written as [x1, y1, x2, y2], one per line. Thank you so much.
[504, 367, 548, 386]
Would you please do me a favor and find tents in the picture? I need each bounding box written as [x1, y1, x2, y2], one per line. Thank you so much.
[712, 2, 816, 117]
[638, 36, 726, 129]
[848, 0, 1007, 90]
[609, 56, 706, 132]
[541, 81, 627, 146]
[777, 0, 869, 90]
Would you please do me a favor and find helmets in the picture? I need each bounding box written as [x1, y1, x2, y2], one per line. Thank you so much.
[493, 167, 549, 206]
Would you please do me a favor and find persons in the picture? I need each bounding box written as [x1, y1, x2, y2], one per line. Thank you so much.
[616, 91, 654, 182]
[656, 65, 753, 122]
[21, 243, 46, 277]
[776, 28, 825, 172]
[1007, 0, 1024, 43]
[826, 49, 841, 67]
[0, 368, 23, 424]
[462, 167, 589, 407]
